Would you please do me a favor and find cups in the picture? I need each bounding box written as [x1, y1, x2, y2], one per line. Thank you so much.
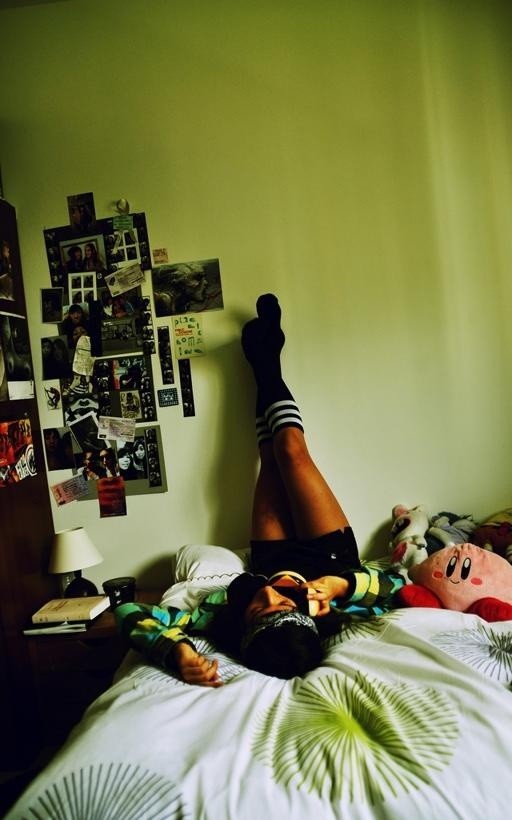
[102, 577, 137, 611]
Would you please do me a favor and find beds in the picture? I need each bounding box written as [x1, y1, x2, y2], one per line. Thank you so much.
[10, 596, 511, 817]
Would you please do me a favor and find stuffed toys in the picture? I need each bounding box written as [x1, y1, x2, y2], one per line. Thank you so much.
[397, 543, 512, 624]
[387, 497, 430, 569]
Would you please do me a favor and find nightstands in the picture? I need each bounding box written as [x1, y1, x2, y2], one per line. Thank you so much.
[27, 588, 162, 754]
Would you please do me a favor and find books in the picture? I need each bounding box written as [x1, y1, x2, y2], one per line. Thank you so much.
[31, 595, 111, 623]
[23, 624, 87, 636]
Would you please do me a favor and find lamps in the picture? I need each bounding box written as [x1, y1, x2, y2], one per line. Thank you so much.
[48, 526, 105, 599]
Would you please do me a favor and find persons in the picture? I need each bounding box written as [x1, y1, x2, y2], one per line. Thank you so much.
[114, 292, 407, 689]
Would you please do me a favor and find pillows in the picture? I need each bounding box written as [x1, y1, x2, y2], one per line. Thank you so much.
[174, 543, 256, 584]
